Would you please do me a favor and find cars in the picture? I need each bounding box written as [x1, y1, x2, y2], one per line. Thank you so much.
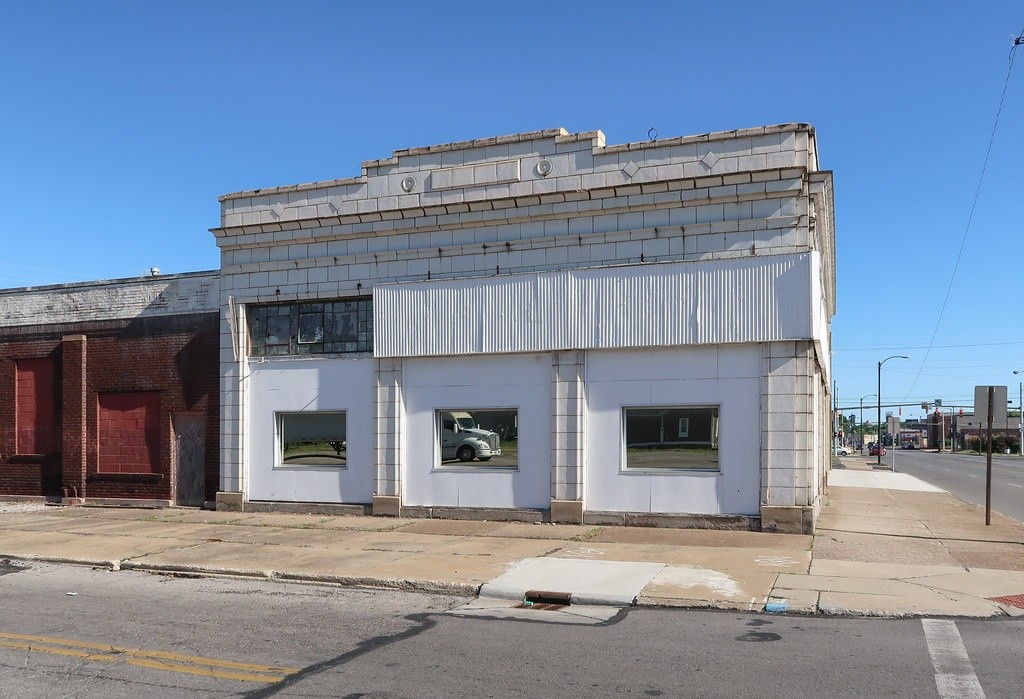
[856, 445, 864, 450]
[869, 444, 887, 456]
[833, 445, 852, 456]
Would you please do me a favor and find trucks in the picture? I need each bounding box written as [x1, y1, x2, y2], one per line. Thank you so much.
[441, 412, 502, 463]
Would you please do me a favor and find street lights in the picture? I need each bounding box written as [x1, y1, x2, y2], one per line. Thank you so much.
[878, 355, 911, 464]
[860, 394, 878, 454]
